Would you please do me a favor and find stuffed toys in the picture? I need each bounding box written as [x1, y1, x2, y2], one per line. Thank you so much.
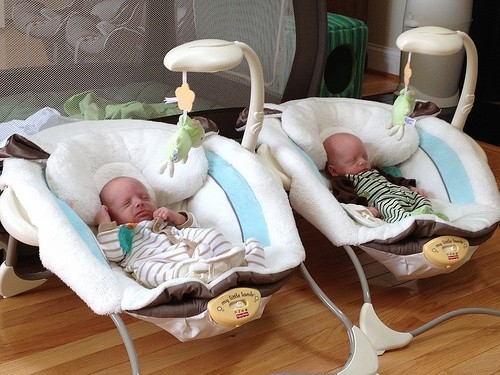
[387, 89, 416, 139]
[159, 115, 205, 178]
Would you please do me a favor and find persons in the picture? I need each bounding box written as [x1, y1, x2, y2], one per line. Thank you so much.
[94, 176, 266, 289]
[323, 132, 449, 227]
